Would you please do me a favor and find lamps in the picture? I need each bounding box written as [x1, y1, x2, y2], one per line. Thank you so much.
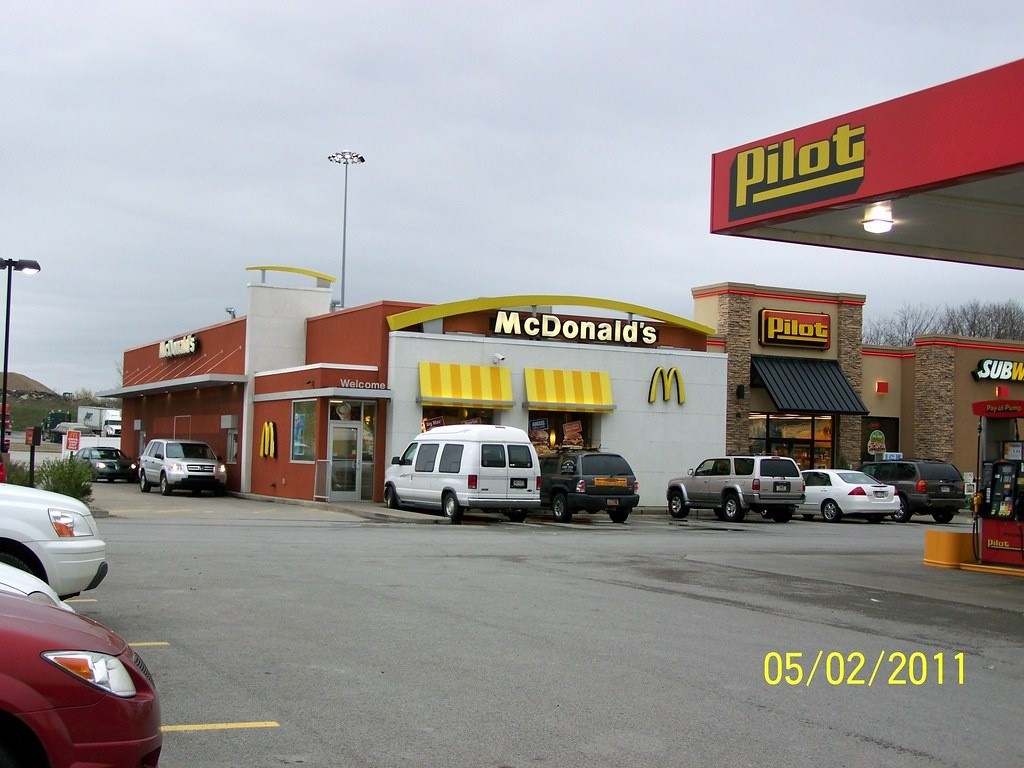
[861, 219, 894, 234]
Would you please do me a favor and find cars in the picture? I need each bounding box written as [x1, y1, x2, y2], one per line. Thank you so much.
[0, 561, 74, 612]
[73, 446, 137, 483]
[41, 410, 96, 444]
[0, 480, 108, 601]
[0, 588, 163, 768]
[761, 469, 901, 523]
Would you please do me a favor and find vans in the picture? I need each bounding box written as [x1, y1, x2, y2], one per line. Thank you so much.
[333, 427, 373, 497]
[382, 424, 542, 520]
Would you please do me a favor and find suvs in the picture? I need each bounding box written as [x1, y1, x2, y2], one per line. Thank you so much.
[848, 457, 970, 523]
[665, 453, 807, 522]
[103, 419, 121, 437]
[139, 438, 228, 496]
[537, 445, 640, 523]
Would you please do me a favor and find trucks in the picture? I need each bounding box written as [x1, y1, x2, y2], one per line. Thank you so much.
[77, 406, 121, 435]
[0, 403, 14, 435]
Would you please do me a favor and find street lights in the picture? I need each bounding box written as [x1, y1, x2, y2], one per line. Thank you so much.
[327, 150, 366, 310]
[0, 258, 42, 456]
